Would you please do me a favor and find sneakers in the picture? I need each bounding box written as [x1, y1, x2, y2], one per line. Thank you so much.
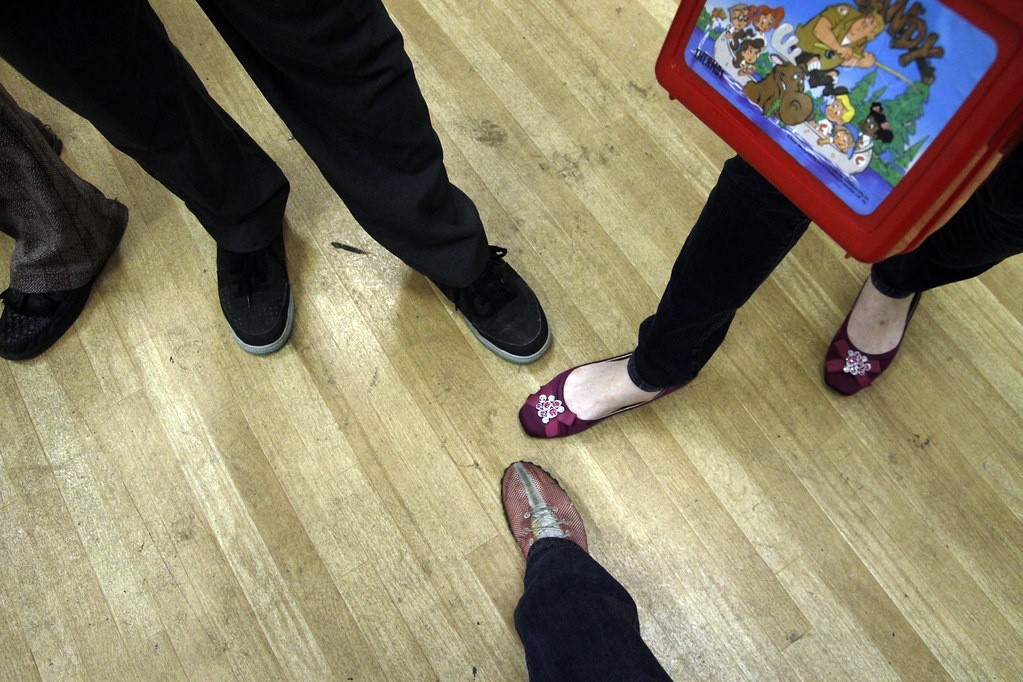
[501, 461, 589, 564]
[426, 245, 552, 363]
[216, 224, 293, 355]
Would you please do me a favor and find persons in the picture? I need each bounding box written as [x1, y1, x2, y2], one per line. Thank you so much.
[499, 461, 674, 682]
[515, 0, 1023, 440]
[0, 0, 548, 367]
[0, 82, 129, 363]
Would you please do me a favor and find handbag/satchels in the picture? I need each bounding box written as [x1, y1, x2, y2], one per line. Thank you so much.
[654, 0, 1023, 264]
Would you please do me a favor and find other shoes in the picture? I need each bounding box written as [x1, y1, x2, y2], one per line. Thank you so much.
[519, 350, 694, 442]
[0, 201, 129, 361]
[824, 274, 924, 397]
